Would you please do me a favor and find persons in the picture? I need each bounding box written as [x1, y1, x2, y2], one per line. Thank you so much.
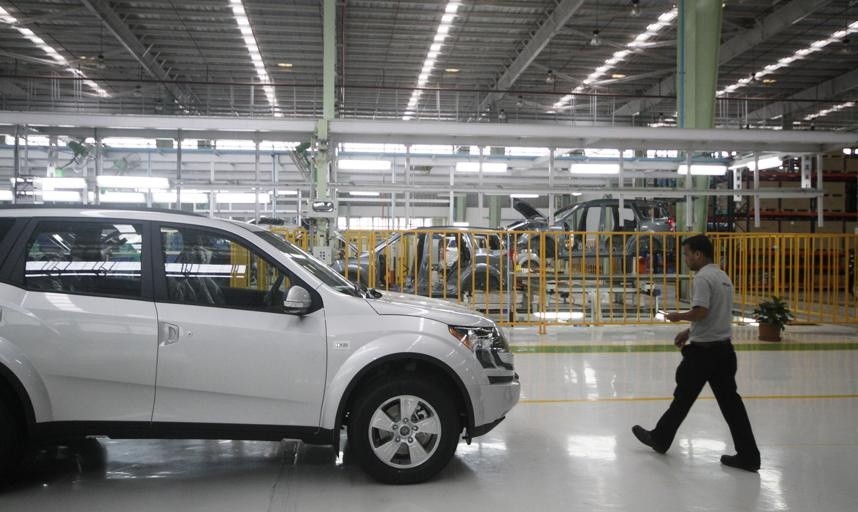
[631, 234, 760, 470]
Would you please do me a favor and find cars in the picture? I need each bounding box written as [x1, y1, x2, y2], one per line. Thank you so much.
[0, 203, 519, 486]
[506, 196, 736, 273]
[327, 223, 542, 299]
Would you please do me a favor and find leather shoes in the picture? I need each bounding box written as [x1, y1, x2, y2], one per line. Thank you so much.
[720, 450, 760, 472]
[631, 425, 672, 454]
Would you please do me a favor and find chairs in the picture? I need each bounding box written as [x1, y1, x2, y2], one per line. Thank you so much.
[164, 242, 226, 306]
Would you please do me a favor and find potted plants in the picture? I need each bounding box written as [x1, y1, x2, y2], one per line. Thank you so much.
[754, 294, 793, 343]
